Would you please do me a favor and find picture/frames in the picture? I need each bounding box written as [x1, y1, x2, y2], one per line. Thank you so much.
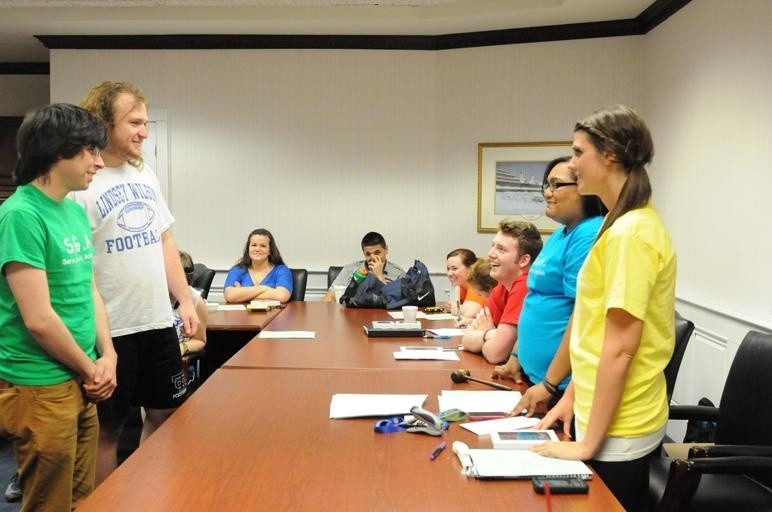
[477, 142, 572, 237]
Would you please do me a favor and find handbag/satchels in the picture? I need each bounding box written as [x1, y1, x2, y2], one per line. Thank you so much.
[345, 259, 436, 307]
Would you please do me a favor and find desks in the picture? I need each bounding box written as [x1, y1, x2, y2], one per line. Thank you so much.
[220, 299, 504, 371]
[204, 301, 284, 332]
[70, 370, 630, 512]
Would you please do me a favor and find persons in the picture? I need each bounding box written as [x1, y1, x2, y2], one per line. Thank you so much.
[508, 105, 677, 511]
[490, 156, 608, 441]
[223, 229, 293, 304]
[0, 101, 119, 512]
[139, 250, 209, 424]
[454, 257, 498, 329]
[446, 249, 488, 319]
[64, 80, 200, 466]
[462, 219, 543, 365]
[322, 231, 406, 301]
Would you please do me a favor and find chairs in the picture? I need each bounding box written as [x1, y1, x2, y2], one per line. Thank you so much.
[664, 312, 695, 405]
[656, 330, 772, 512]
[328, 266, 343, 290]
[192, 267, 215, 299]
[287, 268, 307, 301]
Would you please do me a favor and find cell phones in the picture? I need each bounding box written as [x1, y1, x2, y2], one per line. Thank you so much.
[532, 477, 589, 495]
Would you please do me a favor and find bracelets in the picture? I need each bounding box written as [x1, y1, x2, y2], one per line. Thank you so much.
[181, 342, 191, 355]
[510, 352, 518, 359]
[483, 327, 492, 343]
[541, 377, 558, 397]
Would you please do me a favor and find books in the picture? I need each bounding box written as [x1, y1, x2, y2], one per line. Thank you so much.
[363, 325, 426, 337]
[469, 447, 595, 480]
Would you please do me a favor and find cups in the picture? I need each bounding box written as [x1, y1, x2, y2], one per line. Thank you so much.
[401, 305, 418, 321]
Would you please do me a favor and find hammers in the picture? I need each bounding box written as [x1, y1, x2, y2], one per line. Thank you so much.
[451, 369, 513, 391]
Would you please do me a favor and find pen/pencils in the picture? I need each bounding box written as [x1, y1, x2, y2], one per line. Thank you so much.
[422, 336, 452, 339]
[468, 415, 506, 421]
[431, 441, 447, 460]
[457, 301, 462, 328]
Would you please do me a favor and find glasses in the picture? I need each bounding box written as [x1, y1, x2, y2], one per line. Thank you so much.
[541, 182, 577, 191]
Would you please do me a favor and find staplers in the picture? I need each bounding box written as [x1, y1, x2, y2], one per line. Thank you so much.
[405, 406, 443, 436]
